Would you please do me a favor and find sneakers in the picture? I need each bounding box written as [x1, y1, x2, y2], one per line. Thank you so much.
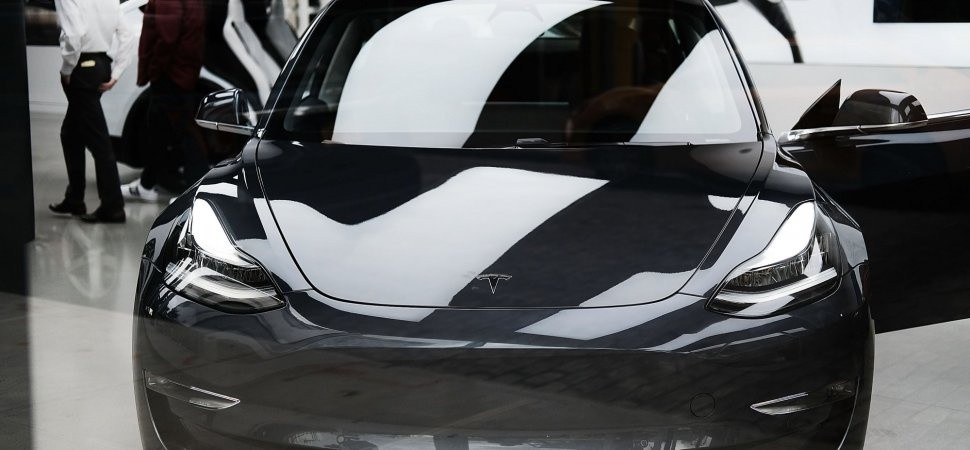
[120, 178, 159, 201]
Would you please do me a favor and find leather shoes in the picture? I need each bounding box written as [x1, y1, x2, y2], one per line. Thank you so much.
[49, 197, 88, 217]
[80, 203, 126, 224]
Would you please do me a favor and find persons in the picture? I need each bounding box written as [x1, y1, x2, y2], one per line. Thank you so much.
[120, 0, 229, 201]
[48, 0, 130, 222]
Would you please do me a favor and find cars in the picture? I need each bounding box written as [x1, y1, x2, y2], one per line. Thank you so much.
[131, 1, 969, 450]
